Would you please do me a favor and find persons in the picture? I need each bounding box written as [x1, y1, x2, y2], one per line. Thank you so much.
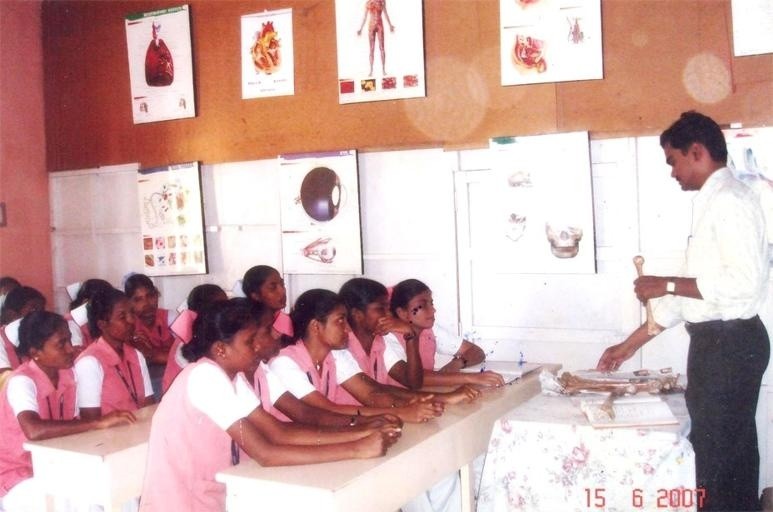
[356, 0, 396, 77]
[2, 274, 174, 499]
[141, 265, 504, 509]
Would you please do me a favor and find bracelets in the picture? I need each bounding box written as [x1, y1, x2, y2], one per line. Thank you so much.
[594, 109, 771, 512]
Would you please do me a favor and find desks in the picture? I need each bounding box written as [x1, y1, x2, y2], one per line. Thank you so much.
[23, 402, 158, 512]
[214, 360, 563, 512]
[476, 391, 698, 512]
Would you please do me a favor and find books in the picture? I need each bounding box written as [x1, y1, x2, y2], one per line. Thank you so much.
[574, 391, 680, 429]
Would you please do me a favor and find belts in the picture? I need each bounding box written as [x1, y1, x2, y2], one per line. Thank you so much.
[684, 318, 756, 337]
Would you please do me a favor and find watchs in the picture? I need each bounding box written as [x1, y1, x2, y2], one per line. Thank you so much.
[664, 276, 675, 296]
